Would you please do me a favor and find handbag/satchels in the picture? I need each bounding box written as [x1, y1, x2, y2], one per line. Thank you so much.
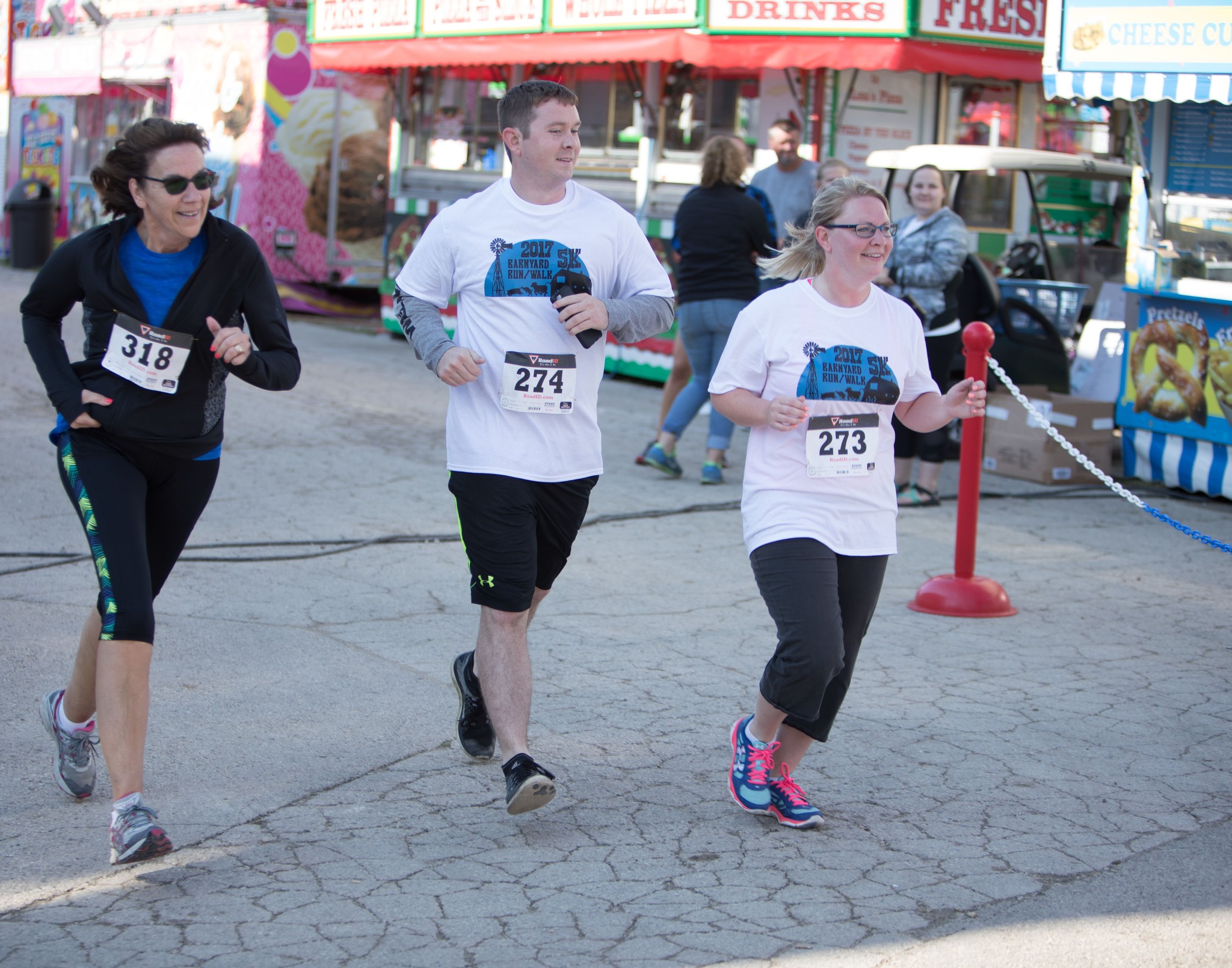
[949, 254, 998, 325]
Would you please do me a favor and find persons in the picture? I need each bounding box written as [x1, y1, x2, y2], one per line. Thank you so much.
[639, 118, 967, 507]
[21, 116, 302, 866]
[391, 79, 673, 808]
[706, 179, 987, 829]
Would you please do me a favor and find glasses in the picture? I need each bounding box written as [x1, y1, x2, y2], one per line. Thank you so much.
[140, 169, 216, 195]
[823, 223, 897, 238]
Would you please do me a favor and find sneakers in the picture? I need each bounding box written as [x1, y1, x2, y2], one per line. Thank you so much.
[643, 443, 684, 477]
[39, 689, 100, 800]
[108, 800, 172, 865]
[702, 461, 722, 484]
[506, 756, 556, 816]
[727, 714, 781, 813]
[767, 762, 825, 827]
[454, 652, 497, 759]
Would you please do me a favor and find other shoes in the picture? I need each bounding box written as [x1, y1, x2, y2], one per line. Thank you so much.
[634, 442, 655, 464]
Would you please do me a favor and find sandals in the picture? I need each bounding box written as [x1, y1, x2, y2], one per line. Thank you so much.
[894, 482, 940, 508]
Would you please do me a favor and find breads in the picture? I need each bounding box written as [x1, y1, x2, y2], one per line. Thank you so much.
[1131, 319, 1210, 427]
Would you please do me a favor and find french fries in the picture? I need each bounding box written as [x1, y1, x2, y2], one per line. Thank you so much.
[1206, 338, 1232, 410]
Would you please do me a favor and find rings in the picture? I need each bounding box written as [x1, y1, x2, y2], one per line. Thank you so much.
[235, 344, 242, 355]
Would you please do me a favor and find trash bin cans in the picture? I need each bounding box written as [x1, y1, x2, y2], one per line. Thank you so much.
[4, 179, 57, 269]
